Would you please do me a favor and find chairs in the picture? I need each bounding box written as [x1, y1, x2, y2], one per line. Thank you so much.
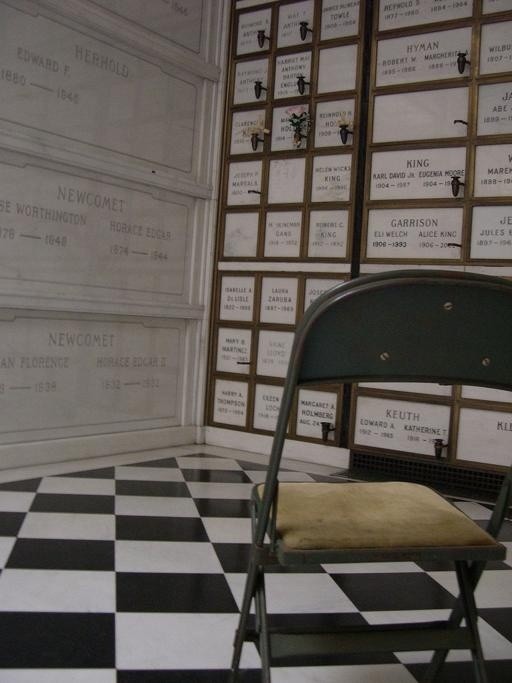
[230, 268, 511, 683]
